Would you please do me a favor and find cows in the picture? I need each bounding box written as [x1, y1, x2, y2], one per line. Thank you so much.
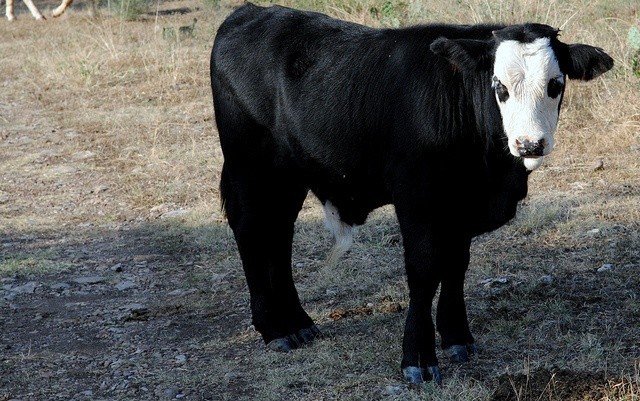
[210, 0, 615, 391]
